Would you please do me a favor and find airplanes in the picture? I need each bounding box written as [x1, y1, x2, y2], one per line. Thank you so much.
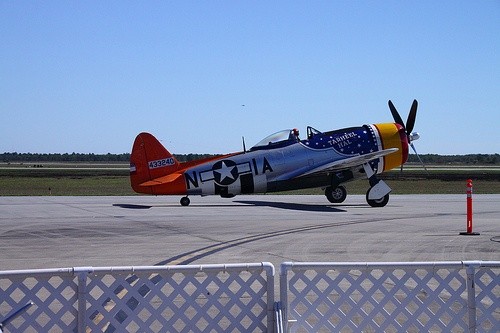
[130, 100, 430, 209]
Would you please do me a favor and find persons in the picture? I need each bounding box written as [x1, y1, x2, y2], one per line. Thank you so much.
[293, 128, 302, 142]
